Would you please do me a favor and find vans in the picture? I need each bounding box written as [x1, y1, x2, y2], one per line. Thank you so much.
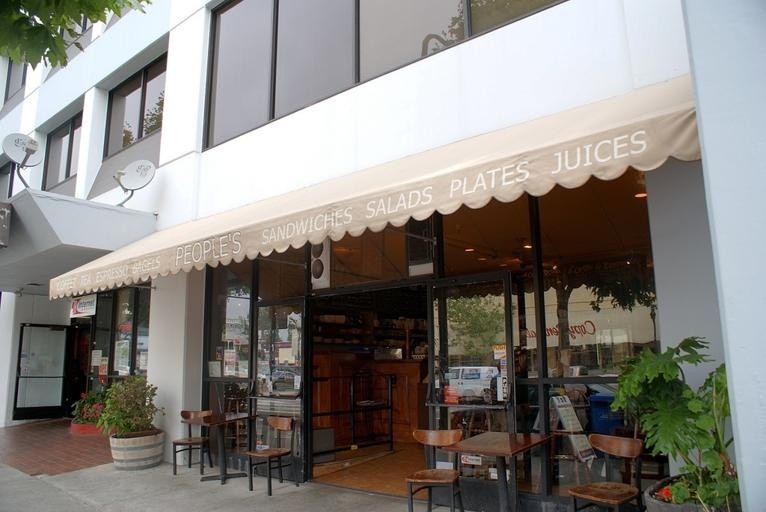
[435, 366, 498, 404]
[570, 366, 588, 377]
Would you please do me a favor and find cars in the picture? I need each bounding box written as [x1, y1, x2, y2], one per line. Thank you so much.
[266, 370, 299, 391]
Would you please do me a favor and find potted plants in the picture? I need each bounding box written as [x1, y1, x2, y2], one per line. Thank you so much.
[608, 332, 741, 512]
[97, 375, 167, 470]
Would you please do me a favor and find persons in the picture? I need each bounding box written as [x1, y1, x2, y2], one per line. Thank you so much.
[546, 346, 590, 434]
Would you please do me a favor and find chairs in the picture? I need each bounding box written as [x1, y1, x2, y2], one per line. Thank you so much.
[245, 415, 300, 495]
[172, 409, 214, 474]
[404, 424, 467, 510]
[565, 431, 644, 512]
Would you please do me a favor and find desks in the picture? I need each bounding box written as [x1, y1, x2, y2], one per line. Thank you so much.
[438, 424, 556, 510]
[182, 411, 257, 485]
